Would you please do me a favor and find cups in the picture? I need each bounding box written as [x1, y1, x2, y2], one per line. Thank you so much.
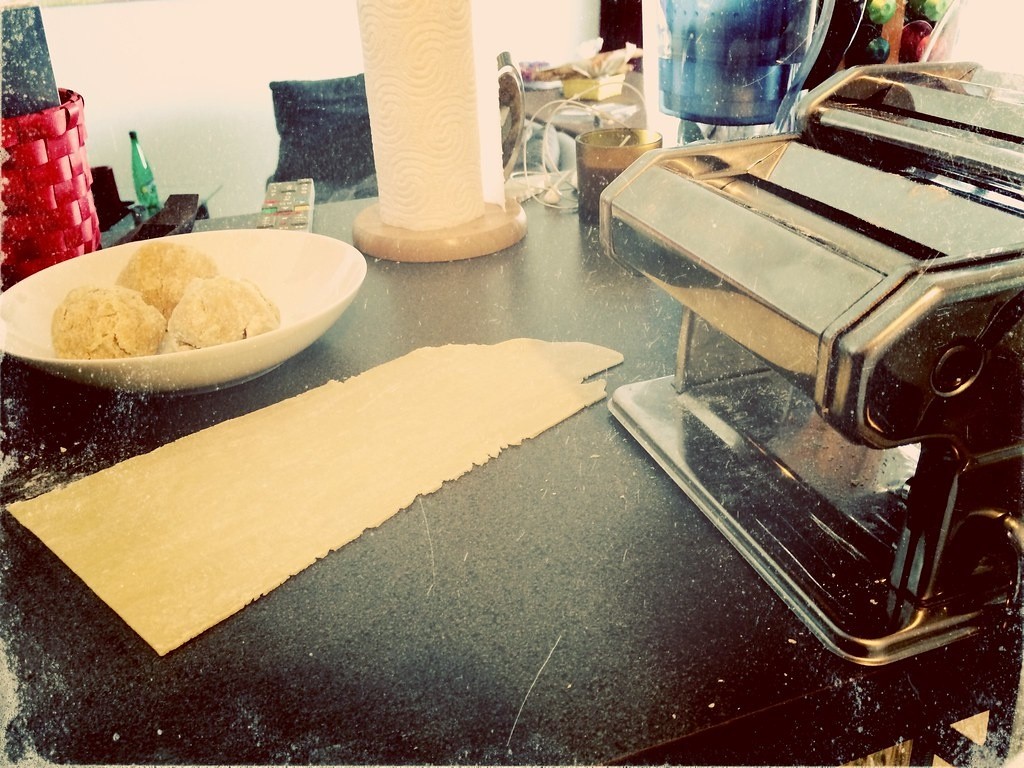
[576, 129, 665, 225]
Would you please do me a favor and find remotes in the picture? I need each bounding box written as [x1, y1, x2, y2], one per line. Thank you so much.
[257, 178, 316, 234]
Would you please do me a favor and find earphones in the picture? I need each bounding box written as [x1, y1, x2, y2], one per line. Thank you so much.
[545, 172, 573, 205]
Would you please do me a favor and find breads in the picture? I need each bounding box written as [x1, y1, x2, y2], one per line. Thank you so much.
[115, 241, 220, 321]
[167, 269, 280, 350]
[51, 287, 165, 358]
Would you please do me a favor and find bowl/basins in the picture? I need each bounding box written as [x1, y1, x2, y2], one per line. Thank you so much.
[0, 229, 370, 392]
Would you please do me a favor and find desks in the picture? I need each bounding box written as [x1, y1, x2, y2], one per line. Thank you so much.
[523, 71, 647, 139]
[1, 165, 1024, 768]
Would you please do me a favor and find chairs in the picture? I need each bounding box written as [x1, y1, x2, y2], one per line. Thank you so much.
[270, 74, 378, 206]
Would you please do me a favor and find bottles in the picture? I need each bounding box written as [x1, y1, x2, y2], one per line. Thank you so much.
[128, 130, 158, 210]
